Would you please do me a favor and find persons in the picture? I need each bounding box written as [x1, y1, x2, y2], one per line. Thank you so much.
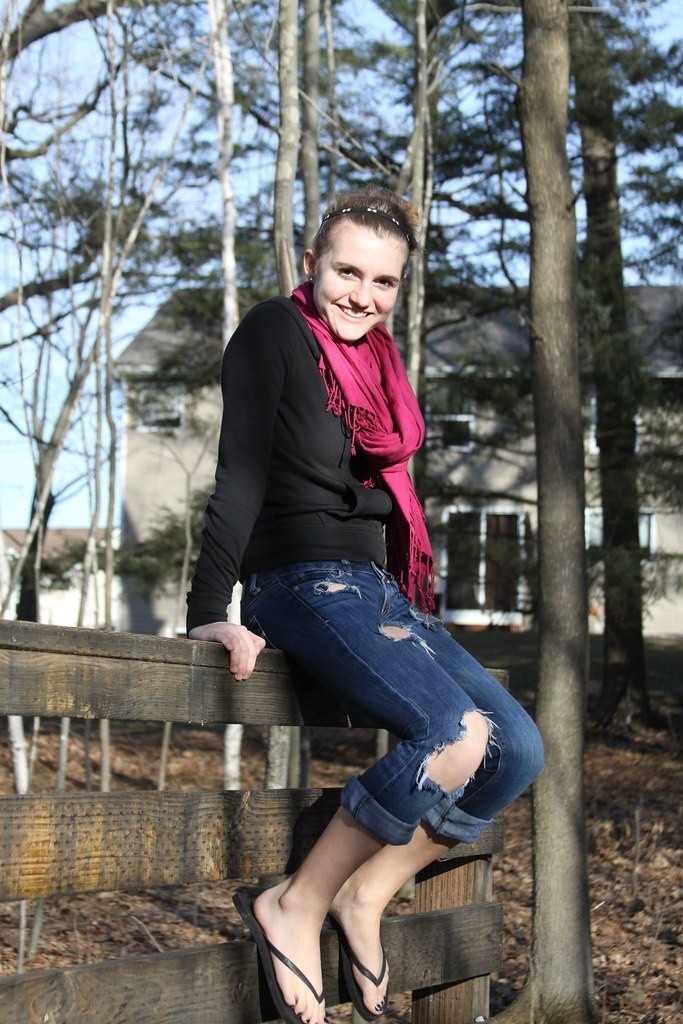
[182, 184, 546, 1024]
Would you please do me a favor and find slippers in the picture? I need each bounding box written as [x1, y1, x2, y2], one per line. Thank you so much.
[338, 923, 390, 1021]
[231, 884, 330, 1024]
